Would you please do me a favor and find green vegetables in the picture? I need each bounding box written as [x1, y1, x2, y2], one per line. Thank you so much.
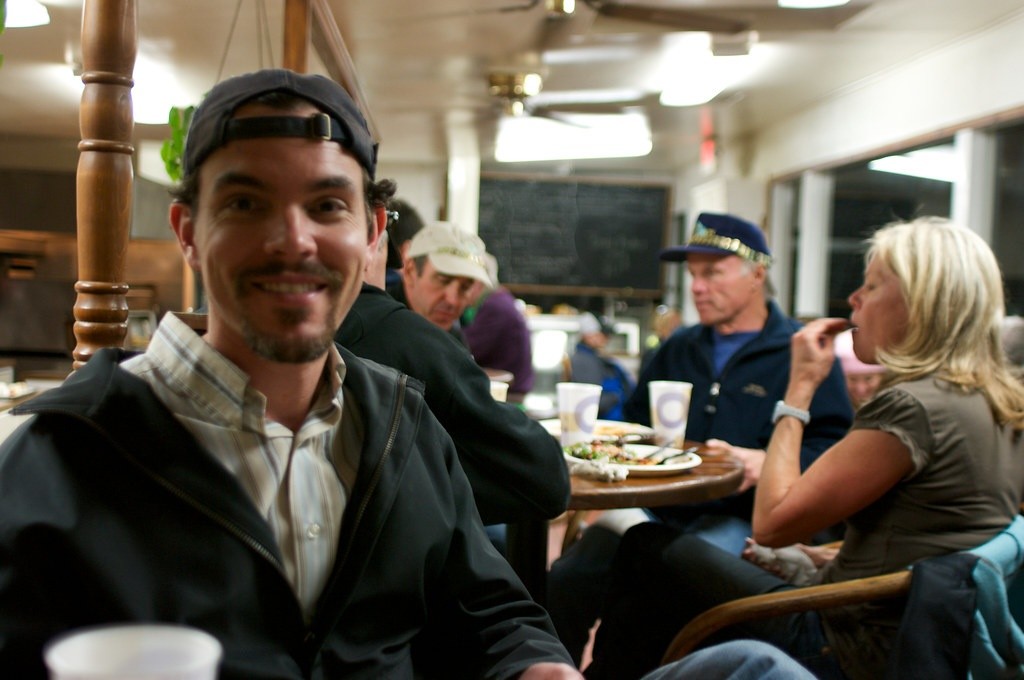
[566, 441, 627, 463]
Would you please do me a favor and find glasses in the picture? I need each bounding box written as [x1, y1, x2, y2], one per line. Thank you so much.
[385, 209, 398, 228]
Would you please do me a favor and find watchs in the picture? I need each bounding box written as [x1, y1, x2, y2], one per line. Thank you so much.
[772, 401, 810, 425]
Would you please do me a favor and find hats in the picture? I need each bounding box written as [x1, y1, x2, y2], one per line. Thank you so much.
[478, 252, 498, 290]
[659, 213, 773, 270]
[183, 68, 375, 180]
[404, 220, 492, 289]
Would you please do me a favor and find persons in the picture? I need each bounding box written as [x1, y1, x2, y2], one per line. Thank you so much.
[0, 68, 585, 679]
[599, 215, 1023, 680]
[551, 209, 881, 644]
[337, 199, 573, 547]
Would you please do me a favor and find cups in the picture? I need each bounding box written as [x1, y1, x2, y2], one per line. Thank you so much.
[558, 382, 602, 434]
[44, 625, 222, 680]
[490, 380, 509, 402]
[649, 380, 693, 450]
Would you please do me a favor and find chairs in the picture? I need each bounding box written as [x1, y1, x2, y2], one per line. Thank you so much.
[661, 507, 1024, 673]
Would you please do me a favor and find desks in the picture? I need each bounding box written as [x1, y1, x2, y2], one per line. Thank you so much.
[538, 416, 747, 509]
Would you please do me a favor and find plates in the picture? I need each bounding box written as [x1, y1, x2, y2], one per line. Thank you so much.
[0, 386, 38, 400]
[536, 419, 653, 439]
[563, 445, 703, 476]
